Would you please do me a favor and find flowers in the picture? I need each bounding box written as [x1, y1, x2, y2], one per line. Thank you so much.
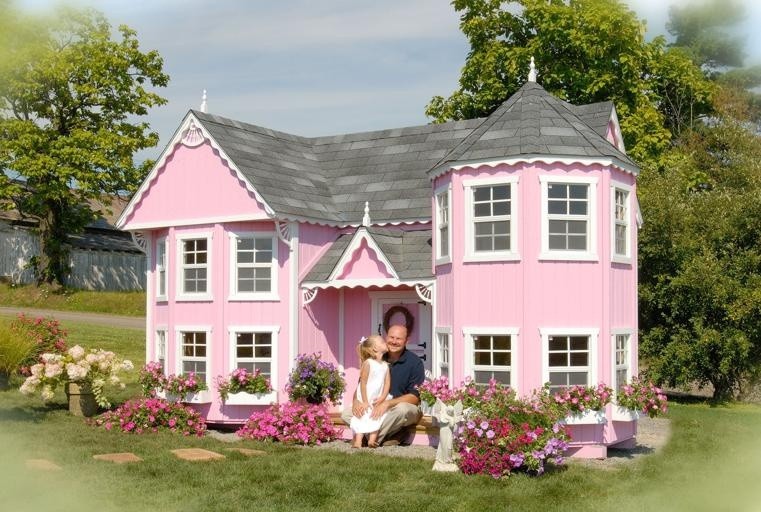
[138, 360, 208, 400]
[552, 381, 614, 414]
[617, 376, 668, 418]
[453, 378, 572, 482]
[409, 373, 483, 409]
[18, 343, 135, 409]
[284, 349, 347, 406]
[212, 367, 274, 400]
[235, 399, 345, 447]
[86, 397, 208, 438]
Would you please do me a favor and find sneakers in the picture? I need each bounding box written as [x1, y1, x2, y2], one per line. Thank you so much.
[381, 429, 410, 446]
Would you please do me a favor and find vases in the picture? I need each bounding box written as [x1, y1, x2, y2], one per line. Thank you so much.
[418, 400, 469, 471]
[65, 381, 101, 416]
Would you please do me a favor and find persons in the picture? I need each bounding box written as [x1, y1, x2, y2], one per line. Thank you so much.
[351, 324, 426, 446]
[349, 335, 391, 450]
